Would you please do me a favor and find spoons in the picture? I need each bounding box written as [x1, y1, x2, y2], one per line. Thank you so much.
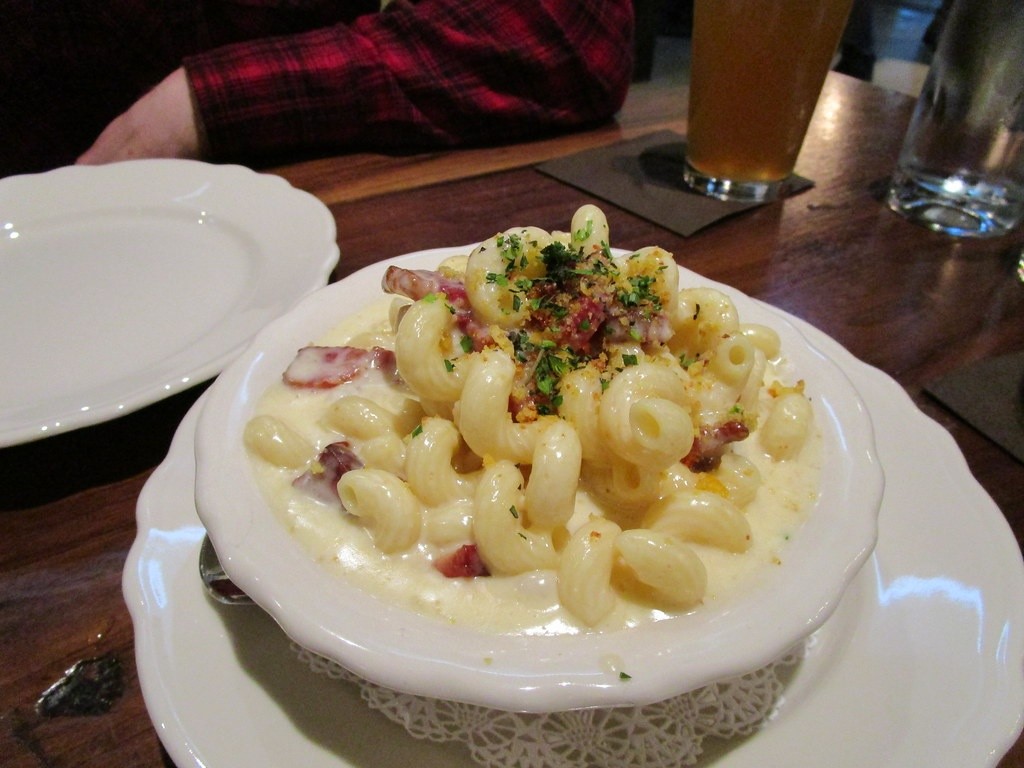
[198, 529, 258, 608]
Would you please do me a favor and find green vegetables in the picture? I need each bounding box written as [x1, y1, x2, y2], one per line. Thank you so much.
[421, 217, 744, 517]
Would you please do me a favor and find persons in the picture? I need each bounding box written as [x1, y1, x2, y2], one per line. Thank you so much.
[0, 0, 640, 184]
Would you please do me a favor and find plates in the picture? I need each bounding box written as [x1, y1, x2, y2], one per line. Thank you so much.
[192, 234, 888, 713]
[0, 158, 341, 450]
[119, 282, 1024, 768]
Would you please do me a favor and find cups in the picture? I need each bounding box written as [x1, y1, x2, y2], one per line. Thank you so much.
[681, 0, 858, 206]
[885, 0, 1024, 238]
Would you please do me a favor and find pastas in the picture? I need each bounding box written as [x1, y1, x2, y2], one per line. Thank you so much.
[243, 204, 808, 630]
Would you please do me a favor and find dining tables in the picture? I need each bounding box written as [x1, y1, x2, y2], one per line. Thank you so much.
[0, 69, 1024, 767]
